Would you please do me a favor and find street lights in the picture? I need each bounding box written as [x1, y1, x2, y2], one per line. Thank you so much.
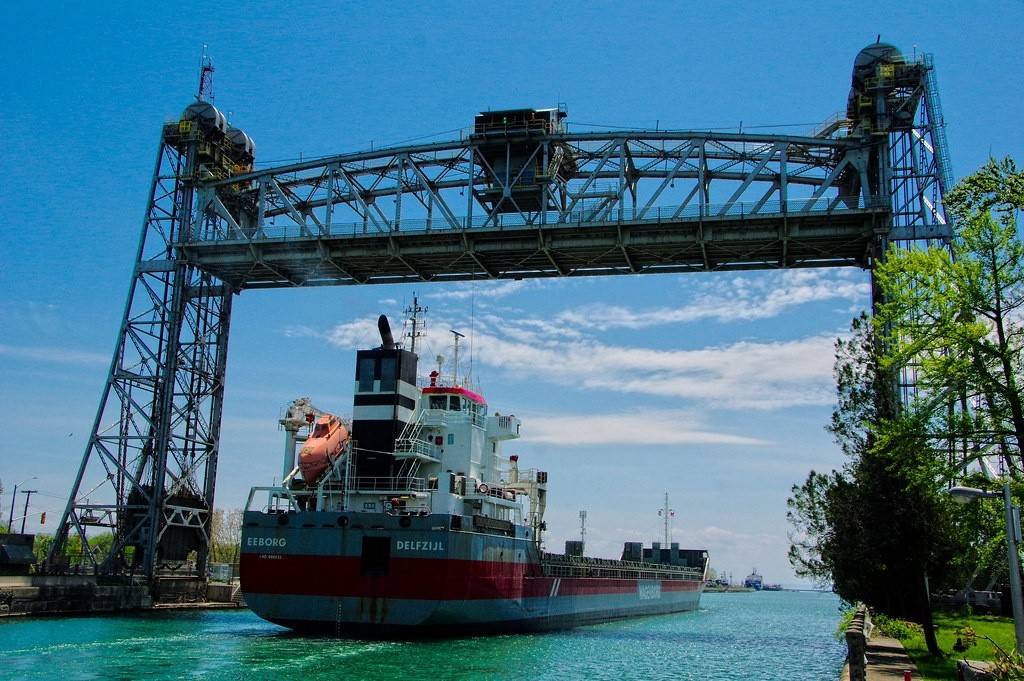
[8, 476, 41, 534]
[949, 483, 1024, 656]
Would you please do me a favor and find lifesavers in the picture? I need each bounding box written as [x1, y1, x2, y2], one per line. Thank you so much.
[479, 483, 488, 493]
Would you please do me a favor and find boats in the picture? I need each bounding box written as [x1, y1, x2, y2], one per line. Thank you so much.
[744, 571, 763, 591]
[239, 293, 710, 639]
[768, 584, 783, 591]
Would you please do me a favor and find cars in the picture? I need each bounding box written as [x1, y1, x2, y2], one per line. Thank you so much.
[705, 579, 713, 586]
[721, 579, 728, 586]
[712, 579, 722, 586]
